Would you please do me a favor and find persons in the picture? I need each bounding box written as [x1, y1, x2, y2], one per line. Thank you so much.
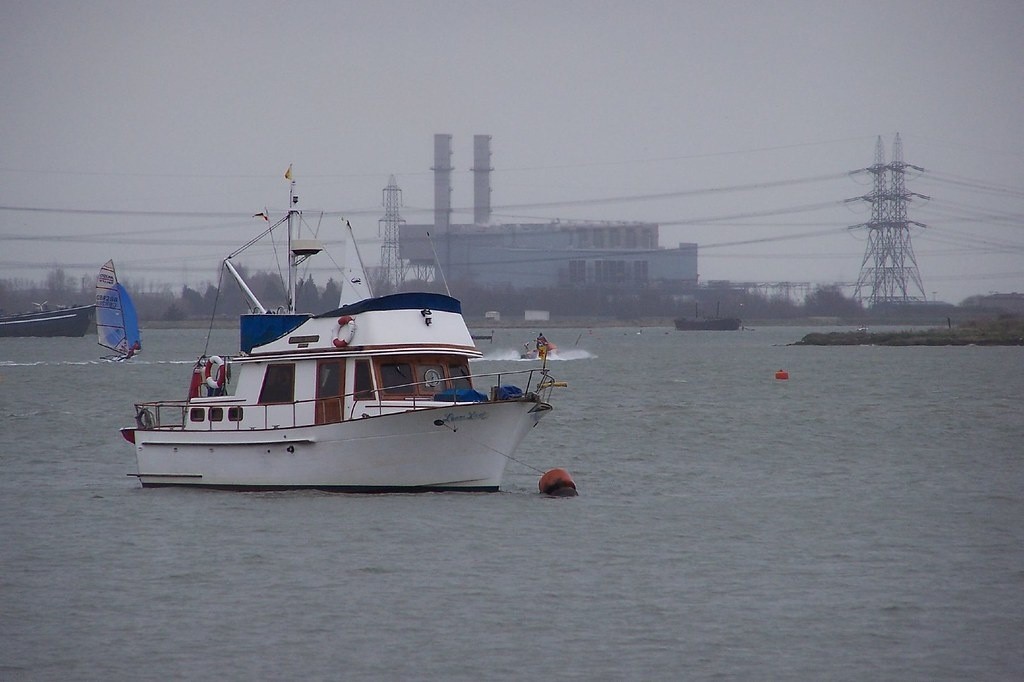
[126, 340, 141, 359]
[536, 333, 549, 349]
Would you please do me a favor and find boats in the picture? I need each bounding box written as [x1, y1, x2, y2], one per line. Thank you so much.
[521, 340, 557, 359]
[0, 303, 96, 338]
[119, 179, 556, 493]
[675, 302, 742, 331]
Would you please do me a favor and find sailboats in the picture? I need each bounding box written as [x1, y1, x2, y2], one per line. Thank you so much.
[95, 259, 144, 363]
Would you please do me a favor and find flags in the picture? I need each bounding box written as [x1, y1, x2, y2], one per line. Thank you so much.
[284, 164, 297, 181]
[539, 346, 546, 358]
[253, 208, 272, 220]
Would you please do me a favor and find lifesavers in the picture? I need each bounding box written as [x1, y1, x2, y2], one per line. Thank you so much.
[330, 315, 357, 347]
[204, 355, 227, 390]
